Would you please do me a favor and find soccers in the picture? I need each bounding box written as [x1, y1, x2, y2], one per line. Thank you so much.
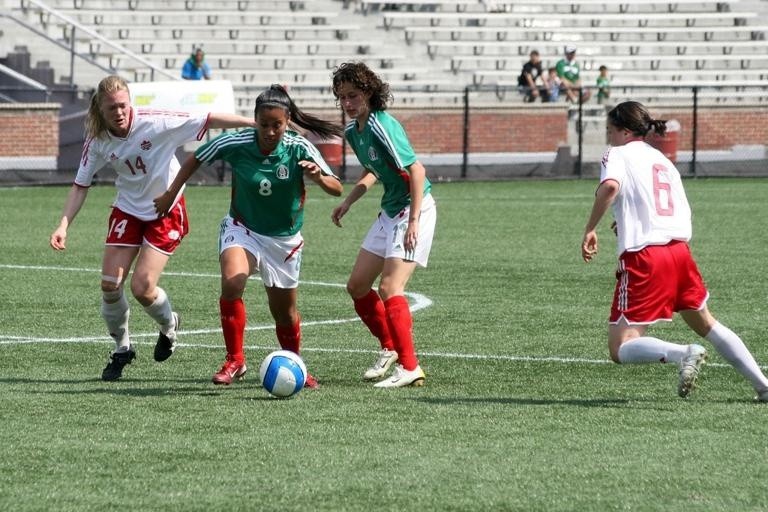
[259, 350, 307, 397]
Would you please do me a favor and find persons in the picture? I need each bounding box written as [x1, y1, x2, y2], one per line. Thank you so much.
[181, 49, 211, 80]
[580, 101, 767, 403]
[50, 76, 257, 382]
[518, 51, 549, 102]
[330, 62, 438, 389]
[545, 69, 566, 103]
[556, 46, 591, 104]
[595, 66, 612, 103]
[153, 85, 344, 390]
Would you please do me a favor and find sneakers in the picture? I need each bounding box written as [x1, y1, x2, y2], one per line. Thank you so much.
[677, 344, 708, 398]
[363, 348, 398, 383]
[304, 374, 319, 389]
[213, 358, 247, 385]
[753, 387, 768, 402]
[102, 344, 136, 381]
[374, 364, 425, 389]
[154, 311, 180, 362]
